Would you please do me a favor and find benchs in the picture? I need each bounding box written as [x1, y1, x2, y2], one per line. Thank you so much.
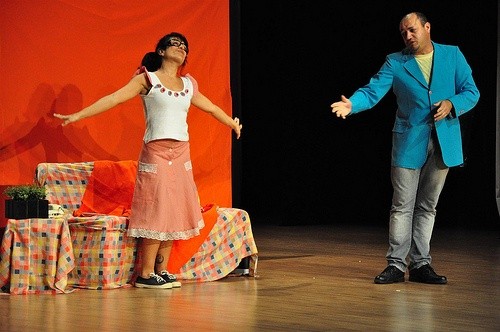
[36, 162, 258, 290]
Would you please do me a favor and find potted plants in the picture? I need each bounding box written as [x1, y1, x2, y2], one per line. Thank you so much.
[4, 185, 49, 219]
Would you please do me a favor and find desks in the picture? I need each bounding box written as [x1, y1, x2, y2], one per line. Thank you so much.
[0, 218, 76, 295]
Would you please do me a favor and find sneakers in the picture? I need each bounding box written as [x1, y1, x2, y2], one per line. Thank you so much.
[135, 270, 182, 290]
[374, 266, 405, 284]
[408, 264, 448, 284]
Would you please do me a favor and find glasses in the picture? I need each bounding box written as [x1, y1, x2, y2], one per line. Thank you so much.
[165, 38, 189, 52]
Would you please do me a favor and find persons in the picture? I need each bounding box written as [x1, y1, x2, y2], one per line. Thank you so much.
[332, 7, 481, 284]
[52, 32, 243, 289]
